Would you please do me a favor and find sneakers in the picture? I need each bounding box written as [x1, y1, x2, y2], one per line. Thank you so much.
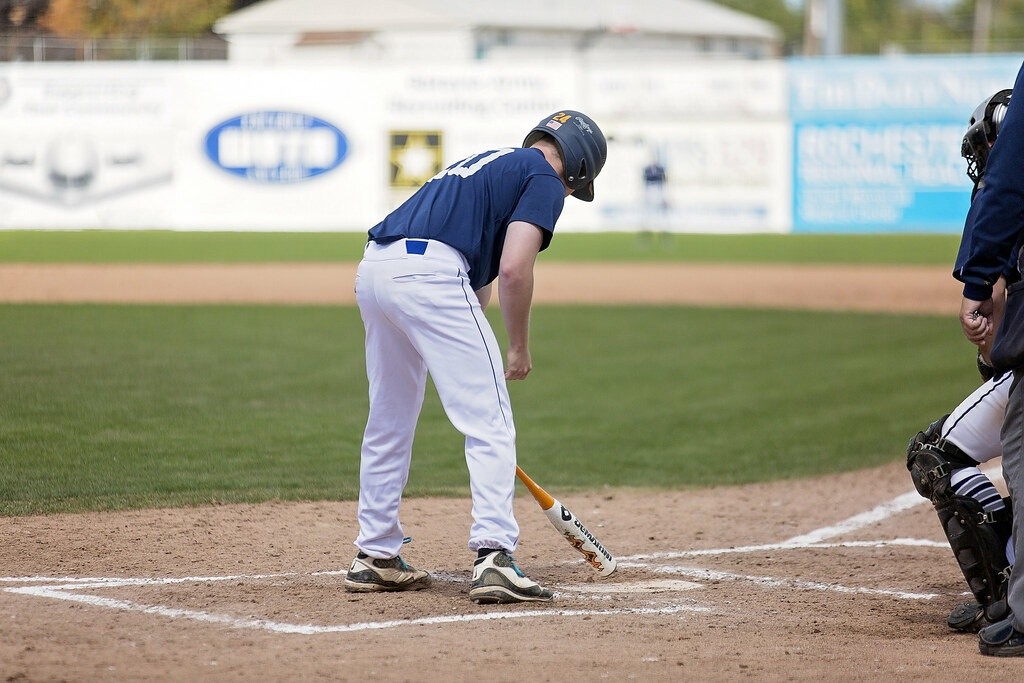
[975, 612, 1023, 659]
[948, 603, 991, 632]
[468, 550, 554, 605]
[344, 548, 432, 593]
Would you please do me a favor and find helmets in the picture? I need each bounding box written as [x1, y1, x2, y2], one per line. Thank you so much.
[962, 87, 1015, 157]
[521, 109, 607, 207]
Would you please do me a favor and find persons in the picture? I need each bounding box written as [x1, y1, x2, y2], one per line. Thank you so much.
[342, 109, 608, 602]
[908, 58, 1024, 654]
[640, 152, 670, 233]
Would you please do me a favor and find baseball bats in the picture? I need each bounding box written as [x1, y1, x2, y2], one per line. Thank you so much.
[516, 464, 617, 578]
